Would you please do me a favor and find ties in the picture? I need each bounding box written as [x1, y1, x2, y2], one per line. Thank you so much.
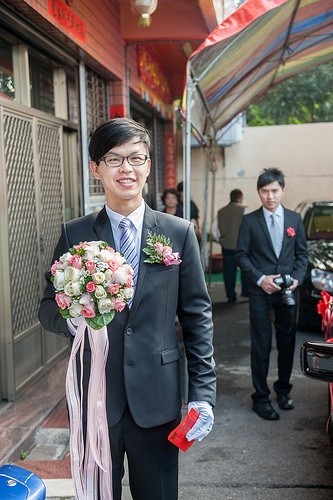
[118, 218, 139, 310]
[269, 214, 283, 259]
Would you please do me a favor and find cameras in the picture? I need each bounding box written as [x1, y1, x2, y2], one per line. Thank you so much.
[272, 274, 298, 308]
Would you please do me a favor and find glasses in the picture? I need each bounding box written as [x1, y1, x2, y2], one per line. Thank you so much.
[97, 152, 150, 167]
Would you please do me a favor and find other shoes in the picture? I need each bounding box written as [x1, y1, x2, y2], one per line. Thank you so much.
[228, 296, 238, 303]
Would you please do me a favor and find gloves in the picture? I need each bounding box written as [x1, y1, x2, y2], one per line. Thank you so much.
[185, 401, 214, 442]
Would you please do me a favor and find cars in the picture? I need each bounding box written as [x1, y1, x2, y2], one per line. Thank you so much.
[299, 340, 333, 446]
[293, 198, 333, 336]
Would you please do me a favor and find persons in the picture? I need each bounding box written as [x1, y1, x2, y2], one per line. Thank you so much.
[162, 182, 256, 304]
[38, 118, 215, 500]
[236, 167, 309, 420]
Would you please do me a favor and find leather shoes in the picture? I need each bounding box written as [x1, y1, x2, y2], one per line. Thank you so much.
[277, 394, 295, 411]
[251, 401, 281, 421]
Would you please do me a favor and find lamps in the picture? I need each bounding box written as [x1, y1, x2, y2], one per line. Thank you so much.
[130, 0, 158, 27]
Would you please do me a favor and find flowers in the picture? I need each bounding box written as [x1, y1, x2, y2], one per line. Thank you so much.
[49, 240, 135, 330]
[286, 227, 295, 237]
[143, 232, 183, 266]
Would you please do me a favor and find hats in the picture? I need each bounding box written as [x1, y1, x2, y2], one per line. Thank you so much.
[177, 181, 182, 191]
